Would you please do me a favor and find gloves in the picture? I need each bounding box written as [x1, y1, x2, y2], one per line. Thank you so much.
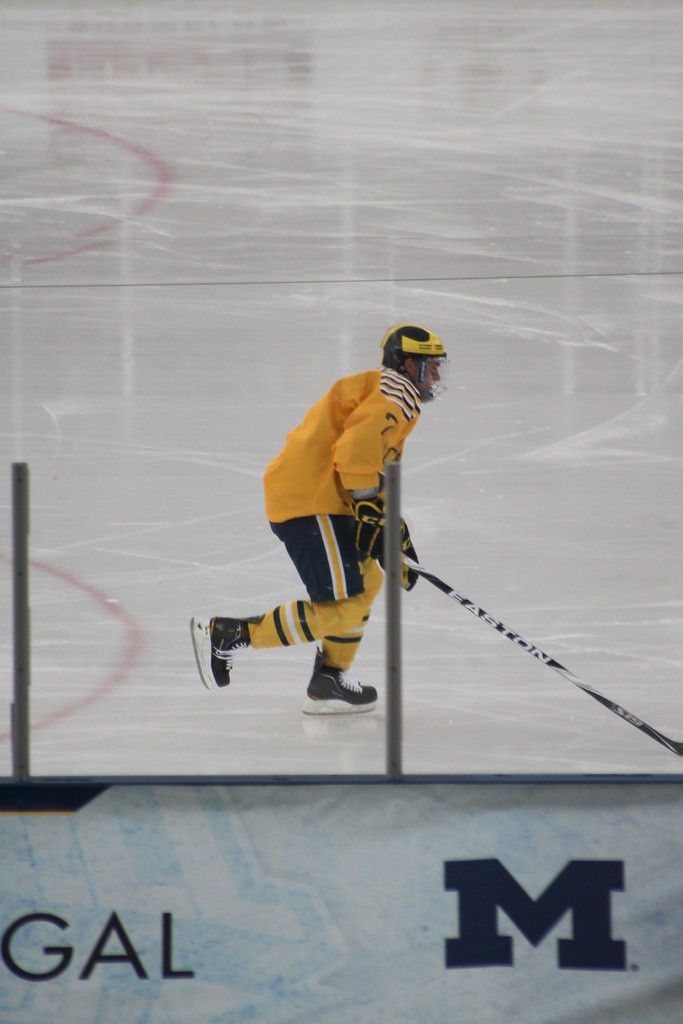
[378, 517, 419, 591]
[349, 497, 387, 559]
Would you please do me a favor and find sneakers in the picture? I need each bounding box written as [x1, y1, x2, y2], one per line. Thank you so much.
[301, 645, 378, 714]
[190, 614, 265, 691]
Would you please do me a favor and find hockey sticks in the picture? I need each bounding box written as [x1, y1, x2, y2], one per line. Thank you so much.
[399, 551, 683, 757]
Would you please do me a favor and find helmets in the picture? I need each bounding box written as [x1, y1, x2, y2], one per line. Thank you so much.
[381, 323, 450, 403]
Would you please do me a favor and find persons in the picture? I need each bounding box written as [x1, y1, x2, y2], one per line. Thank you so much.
[189, 322, 450, 716]
